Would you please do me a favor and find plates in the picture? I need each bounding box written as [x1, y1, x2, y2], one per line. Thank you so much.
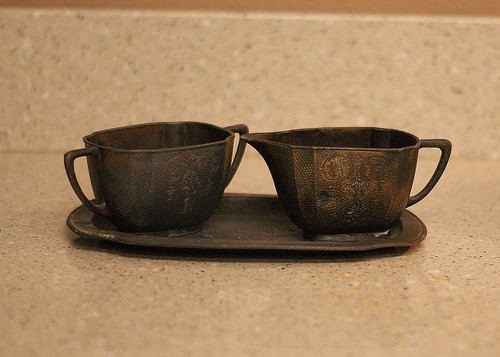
[66, 190, 427, 252]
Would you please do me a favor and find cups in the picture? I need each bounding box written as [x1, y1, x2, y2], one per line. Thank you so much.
[240, 126, 453, 236]
[63, 121, 250, 234]
